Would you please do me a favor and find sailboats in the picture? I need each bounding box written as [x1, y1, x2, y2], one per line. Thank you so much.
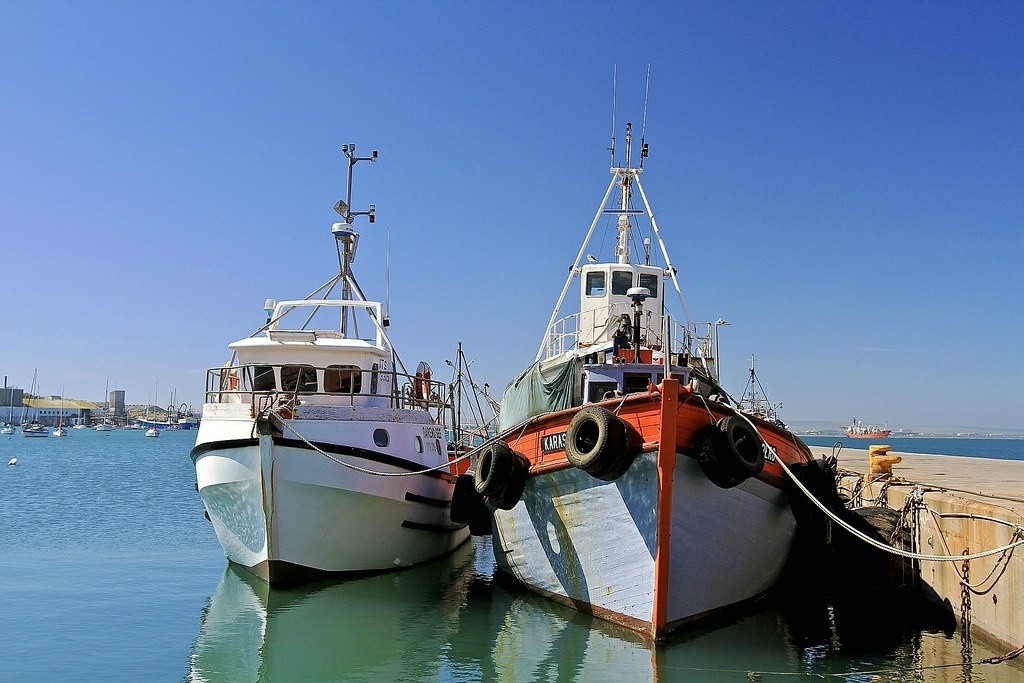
[97, 380, 112, 431]
[52, 380, 68, 436]
[1, 386, 16, 434]
[23, 369, 49, 437]
[146, 380, 160, 437]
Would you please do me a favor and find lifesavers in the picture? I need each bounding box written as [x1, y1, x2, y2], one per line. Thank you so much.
[414, 360, 432, 407]
[221, 361, 237, 391]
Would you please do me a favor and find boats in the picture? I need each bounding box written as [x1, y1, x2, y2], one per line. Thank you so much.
[843, 416, 891, 438]
[138, 416, 192, 428]
[190, 142, 470, 589]
[474, 64, 814, 645]
[8, 458, 17, 466]
